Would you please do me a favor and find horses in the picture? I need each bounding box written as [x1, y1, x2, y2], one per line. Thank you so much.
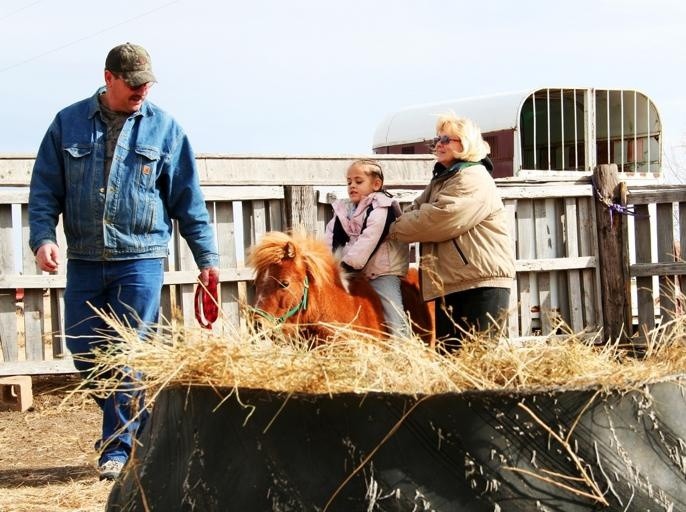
[241, 224, 440, 350]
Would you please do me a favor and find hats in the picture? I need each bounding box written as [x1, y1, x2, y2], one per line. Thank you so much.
[106, 41, 158, 89]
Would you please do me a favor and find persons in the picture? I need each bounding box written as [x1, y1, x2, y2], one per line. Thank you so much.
[325, 158, 412, 340]
[384, 112, 515, 360]
[27, 40, 221, 480]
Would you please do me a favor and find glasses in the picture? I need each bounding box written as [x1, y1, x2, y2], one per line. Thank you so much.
[432, 135, 462, 148]
[111, 73, 149, 91]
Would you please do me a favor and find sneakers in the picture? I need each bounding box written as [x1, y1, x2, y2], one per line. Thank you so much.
[96, 438, 105, 449]
[99, 458, 123, 482]
